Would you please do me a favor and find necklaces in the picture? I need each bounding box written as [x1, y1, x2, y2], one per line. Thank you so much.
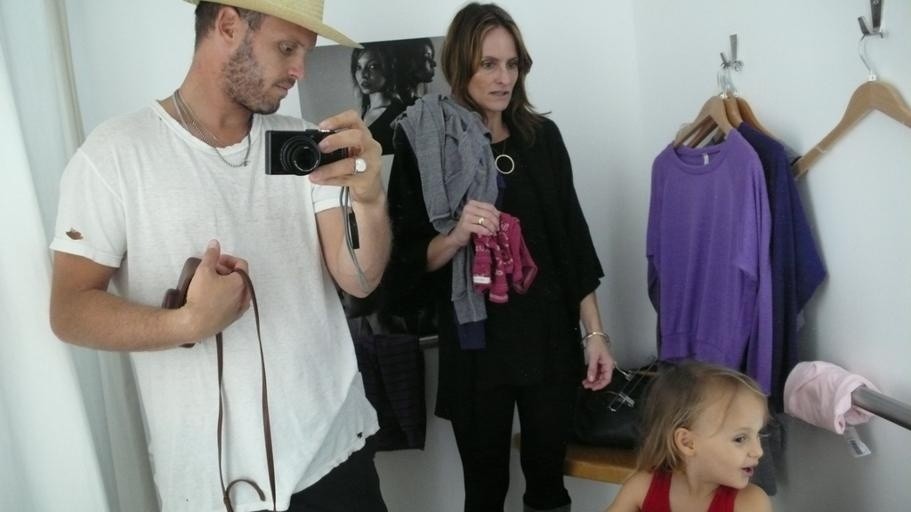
[169, 89, 251, 169]
[484, 117, 518, 177]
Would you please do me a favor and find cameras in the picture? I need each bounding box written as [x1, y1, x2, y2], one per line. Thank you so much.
[265, 129, 349, 176]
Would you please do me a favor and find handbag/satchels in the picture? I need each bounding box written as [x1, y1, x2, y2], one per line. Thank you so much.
[354, 317, 426, 450]
[578, 358, 677, 449]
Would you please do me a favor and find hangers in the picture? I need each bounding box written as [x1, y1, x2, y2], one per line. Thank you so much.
[668, 31, 910, 183]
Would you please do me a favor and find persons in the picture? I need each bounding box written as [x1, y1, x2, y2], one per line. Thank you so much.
[396, 0, 616, 512]
[395, 35, 440, 105]
[330, 276, 429, 453]
[46, 1, 393, 511]
[345, 37, 437, 199]
[605, 359, 775, 512]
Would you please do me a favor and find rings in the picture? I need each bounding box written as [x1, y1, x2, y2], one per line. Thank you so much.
[477, 215, 485, 226]
[352, 152, 366, 177]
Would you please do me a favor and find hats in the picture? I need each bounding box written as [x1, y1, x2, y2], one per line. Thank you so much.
[184, 0, 364, 49]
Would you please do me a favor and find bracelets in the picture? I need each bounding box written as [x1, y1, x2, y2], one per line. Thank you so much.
[577, 330, 613, 353]
